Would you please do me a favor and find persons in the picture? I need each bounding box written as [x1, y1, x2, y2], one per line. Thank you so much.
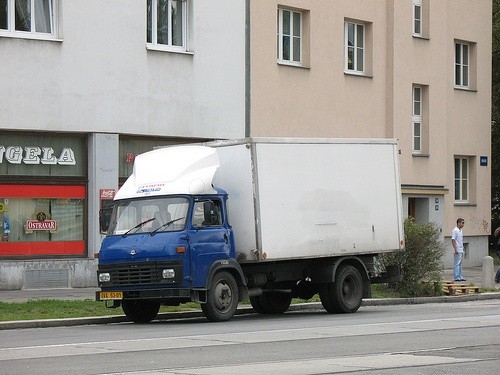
[452, 218, 467, 281]
[494, 227, 500, 283]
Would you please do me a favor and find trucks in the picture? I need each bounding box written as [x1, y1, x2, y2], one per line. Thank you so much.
[93, 136, 407, 324]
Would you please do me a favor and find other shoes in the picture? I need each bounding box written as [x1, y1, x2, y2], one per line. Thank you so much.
[455, 279, 466, 281]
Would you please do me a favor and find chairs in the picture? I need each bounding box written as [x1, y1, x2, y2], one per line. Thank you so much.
[153, 211, 172, 230]
